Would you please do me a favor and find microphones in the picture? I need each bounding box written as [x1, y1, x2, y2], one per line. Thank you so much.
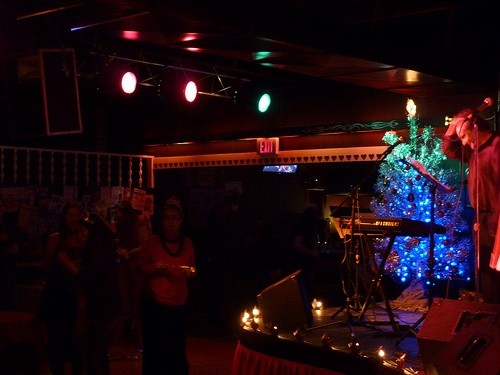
[377, 136, 405, 164]
[464, 97, 494, 122]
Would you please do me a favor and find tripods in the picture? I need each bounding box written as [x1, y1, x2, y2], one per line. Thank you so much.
[305, 158, 458, 345]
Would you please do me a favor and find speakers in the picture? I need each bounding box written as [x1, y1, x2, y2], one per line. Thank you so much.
[417, 297, 500, 375]
[38, 47, 83, 136]
[257, 270, 313, 330]
[433, 312, 500, 375]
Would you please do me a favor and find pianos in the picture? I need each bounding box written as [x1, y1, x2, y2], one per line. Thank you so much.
[338, 217, 447, 235]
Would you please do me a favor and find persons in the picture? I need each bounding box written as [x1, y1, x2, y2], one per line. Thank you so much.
[0, 203, 151, 375]
[135, 198, 196, 375]
[283, 204, 324, 284]
[440, 109, 500, 306]
